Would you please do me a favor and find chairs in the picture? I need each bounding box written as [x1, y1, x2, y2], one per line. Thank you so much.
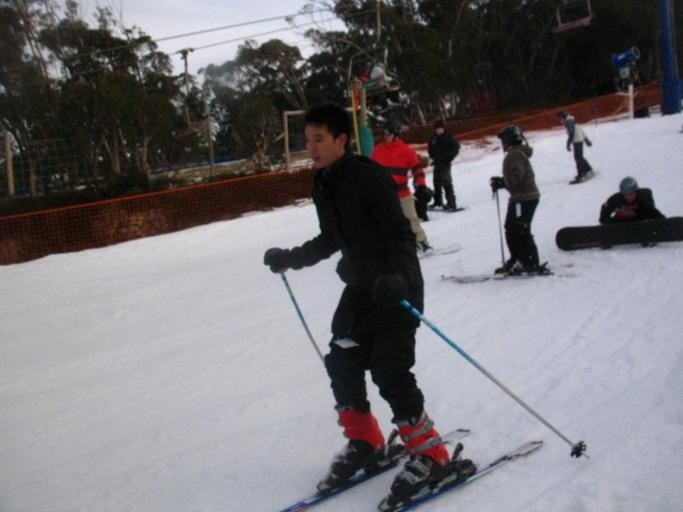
[390, 454, 450, 493]
[326, 439, 386, 486]
[443, 196, 456, 208]
[420, 240, 433, 256]
[510, 256, 539, 274]
[496, 257, 514, 274]
[430, 194, 443, 207]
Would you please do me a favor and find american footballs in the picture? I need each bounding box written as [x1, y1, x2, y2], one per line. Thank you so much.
[417, 243, 459, 259]
[569, 171, 597, 185]
[427, 204, 465, 212]
[556, 216, 683, 251]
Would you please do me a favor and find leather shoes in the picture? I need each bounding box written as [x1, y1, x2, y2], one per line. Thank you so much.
[263, 244, 304, 274]
[490, 177, 504, 191]
[585, 139, 592, 146]
[567, 139, 571, 151]
[374, 268, 414, 308]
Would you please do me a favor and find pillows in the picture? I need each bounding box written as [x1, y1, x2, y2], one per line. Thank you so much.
[620, 178, 638, 194]
[498, 126, 522, 149]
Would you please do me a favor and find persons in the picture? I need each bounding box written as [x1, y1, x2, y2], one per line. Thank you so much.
[487, 125, 543, 276]
[552, 108, 595, 186]
[369, 122, 430, 254]
[429, 122, 459, 211]
[264, 100, 450, 493]
[598, 174, 663, 219]
